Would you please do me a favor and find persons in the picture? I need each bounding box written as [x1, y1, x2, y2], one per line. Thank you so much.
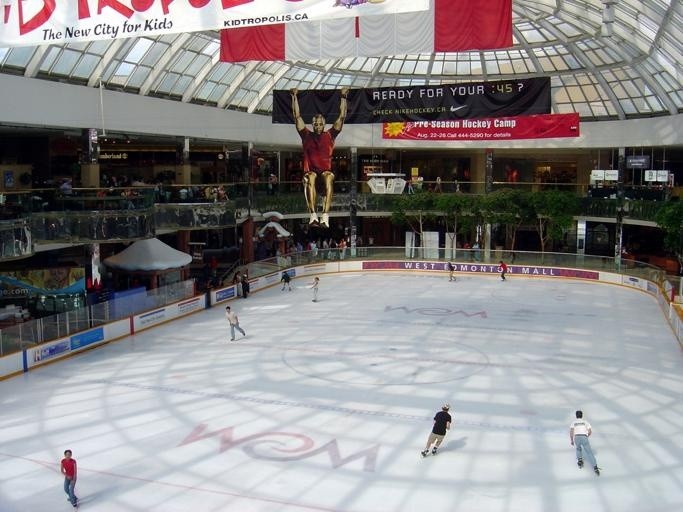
[291, 88, 350, 229]
[195, 257, 251, 300]
[447, 262, 456, 283]
[60, 450, 80, 512]
[280, 271, 292, 291]
[569, 411, 600, 478]
[225, 306, 245, 341]
[309, 277, 320, 303]
[420, 402, 451, 458]
[57, 174, 230, 221]
[463, 240, 482, 264]
[498, 260, 508, 282]
[254, 231, 374, 266]
[265, 173, 280, 196]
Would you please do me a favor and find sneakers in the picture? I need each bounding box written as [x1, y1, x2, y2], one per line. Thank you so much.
[420, 446, 438, 457]
[309, 210, 331, 230]
[577, 456, 601, 476]
